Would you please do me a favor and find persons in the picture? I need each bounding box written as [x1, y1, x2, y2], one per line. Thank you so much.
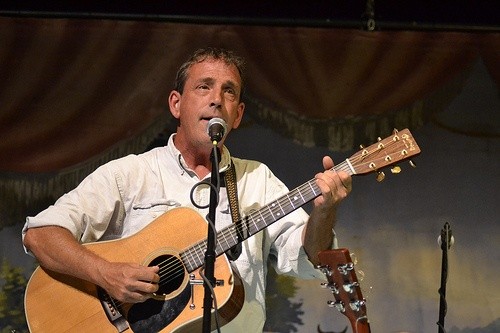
[21, 44, 353, 333]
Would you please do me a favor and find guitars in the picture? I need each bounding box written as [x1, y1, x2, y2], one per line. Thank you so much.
[23, 127, 422, 333]
[317, 248, 372, 333]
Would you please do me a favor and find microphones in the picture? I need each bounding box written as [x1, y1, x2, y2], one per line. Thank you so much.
[437, 223, 454, 247]
[207, 117, 228, 144]
